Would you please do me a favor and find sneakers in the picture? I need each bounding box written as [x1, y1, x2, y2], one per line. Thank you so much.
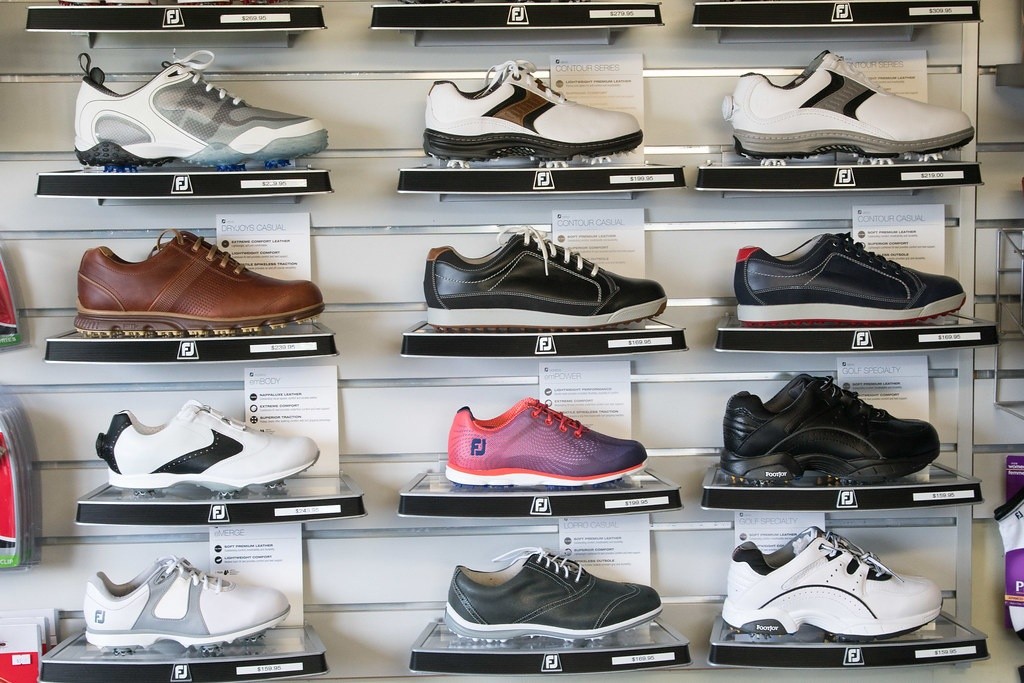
[67, 54, 977, 649]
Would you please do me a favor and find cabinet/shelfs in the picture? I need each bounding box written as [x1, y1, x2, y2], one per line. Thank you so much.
[2, 1, 1024, 681]
